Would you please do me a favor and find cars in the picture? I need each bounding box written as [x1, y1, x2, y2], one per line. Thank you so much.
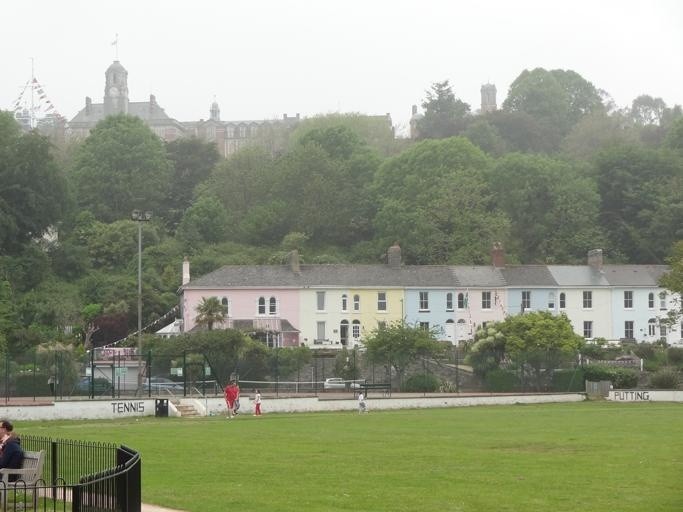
[324, 378, 360, 389]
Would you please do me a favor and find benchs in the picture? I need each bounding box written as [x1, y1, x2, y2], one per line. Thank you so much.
[354, 383, 390, 398]
[0, 448, 46, 512]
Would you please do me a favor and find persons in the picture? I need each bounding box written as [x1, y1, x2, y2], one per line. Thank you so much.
[254, 389, 262, 416]
[0, 419, 23, 482]
[224, 380, 238, 419]
[358, 391, 367, 415]
[234, 382, 240, 415]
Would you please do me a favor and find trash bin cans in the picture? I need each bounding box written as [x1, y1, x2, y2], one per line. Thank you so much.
[155, 399, 168, 417]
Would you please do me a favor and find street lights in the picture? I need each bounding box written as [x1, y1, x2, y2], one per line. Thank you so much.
[130, 209, 152, 395]
